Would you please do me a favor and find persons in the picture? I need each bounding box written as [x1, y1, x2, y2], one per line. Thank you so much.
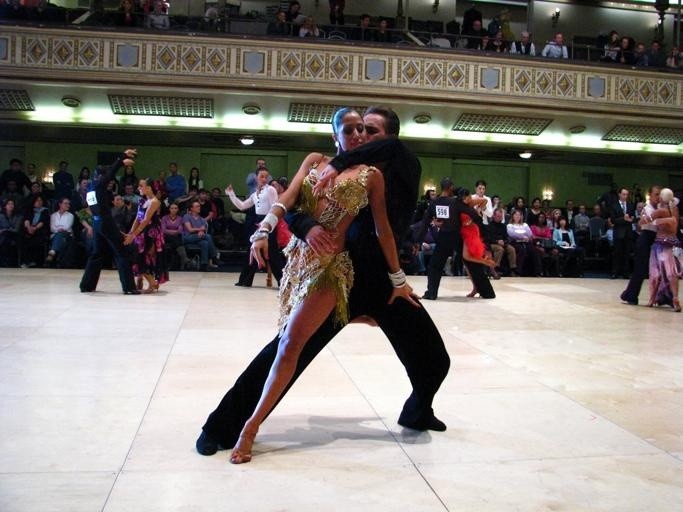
[398, 178, 683, 312]
[1, 1, 569, 59]
[196, 106, 450, 456]
[229, 107, 423, 464]
[1, 150, 294, 295]
[596, 31, 682, 70]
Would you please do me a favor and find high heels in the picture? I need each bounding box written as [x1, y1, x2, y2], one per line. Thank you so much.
[644, 300, 656, 308]
[672, 297, 681, 312]
[467, 288, 481, 298]
[144, 280, 159, 293]
[489, 270, 500, 279]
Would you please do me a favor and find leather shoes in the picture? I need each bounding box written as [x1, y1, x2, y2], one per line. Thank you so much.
[429, 416, 446, 431]
[197, 430, 219, 455]
[124, 289, 140, 294]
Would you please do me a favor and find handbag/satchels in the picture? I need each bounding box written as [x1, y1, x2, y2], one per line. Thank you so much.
[191, 255, 200, 270]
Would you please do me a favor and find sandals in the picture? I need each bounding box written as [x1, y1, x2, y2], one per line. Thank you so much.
[230, 430, 257, 464]
